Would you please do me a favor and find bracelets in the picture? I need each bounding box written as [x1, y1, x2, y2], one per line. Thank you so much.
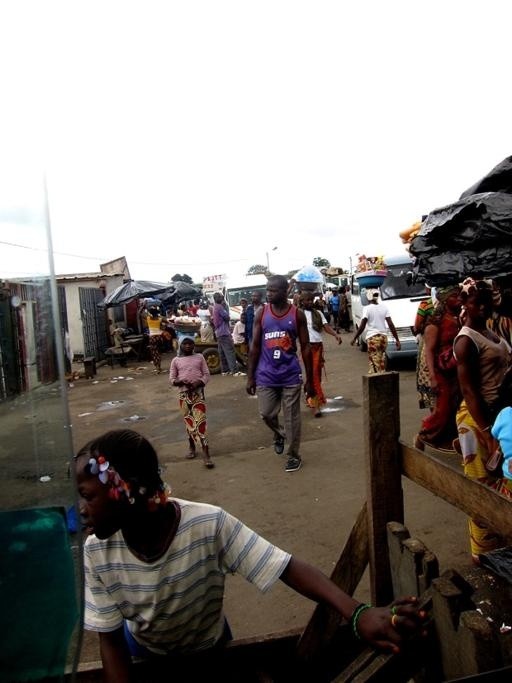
[351, 602, 375, 639]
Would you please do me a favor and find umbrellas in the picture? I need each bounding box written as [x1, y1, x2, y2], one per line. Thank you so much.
[98, 281, 176, 335]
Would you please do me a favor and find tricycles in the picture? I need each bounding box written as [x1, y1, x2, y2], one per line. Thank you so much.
[191, 337, 249, 374]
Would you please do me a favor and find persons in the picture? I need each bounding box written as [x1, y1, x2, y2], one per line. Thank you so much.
[68, 428, 430, 682]
[179, 285, 354, 337]
[412, 277, 512, 581]
[245, 275, 316, 473]
[213, 292, 246, 377]
[351, 286, 401, 376]
[139, 299, 168, 373]
[169, 332, 215, 470]
[286, 279, 342, 417]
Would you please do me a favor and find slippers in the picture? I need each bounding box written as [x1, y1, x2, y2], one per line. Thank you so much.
[186, 451, 198, 459]
[204, 456, 214, 465]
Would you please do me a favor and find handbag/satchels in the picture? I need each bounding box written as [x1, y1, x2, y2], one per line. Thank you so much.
[485, 450, 505, 478]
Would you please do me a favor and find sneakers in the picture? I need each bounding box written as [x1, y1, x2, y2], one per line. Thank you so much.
[285, 458, 302, 472]
[274, 425, 284, 455]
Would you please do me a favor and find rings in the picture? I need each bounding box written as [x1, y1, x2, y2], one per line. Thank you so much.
[391, 615, 398, 627]
[391, 606, 401, 614]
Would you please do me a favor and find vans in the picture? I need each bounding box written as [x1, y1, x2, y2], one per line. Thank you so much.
[285, 271, 352, 325]
[352, 256, 446, 369]
[201, 273, 272, 336]
